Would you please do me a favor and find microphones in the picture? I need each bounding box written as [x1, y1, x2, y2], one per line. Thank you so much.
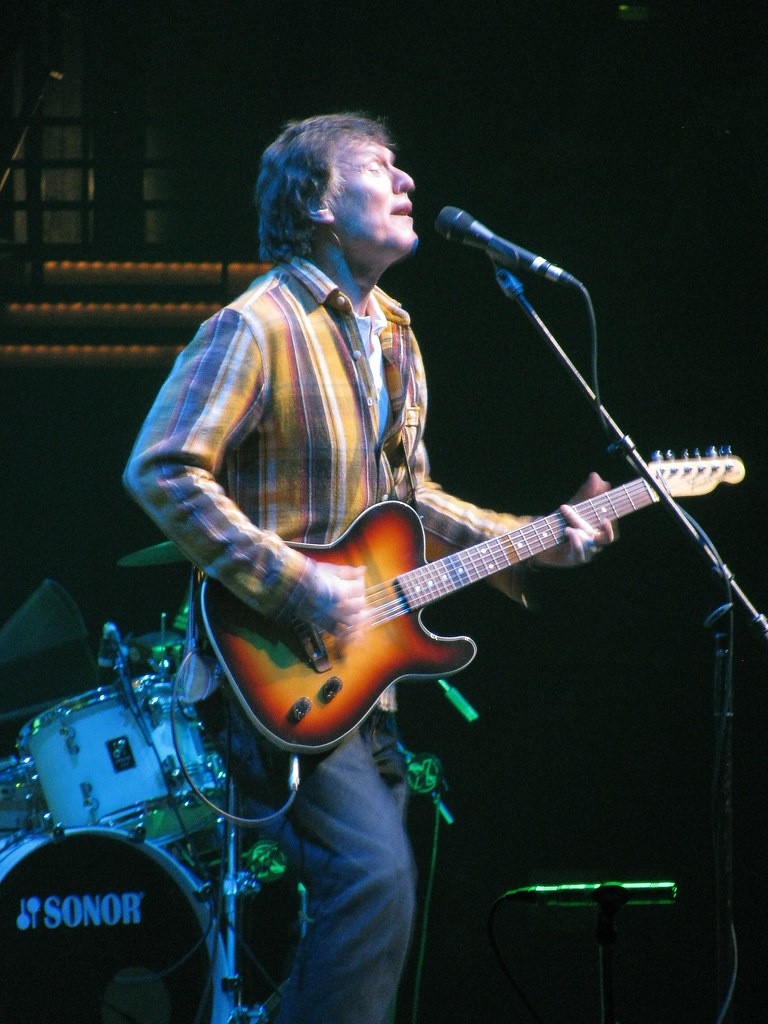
[96, 623, 117, 687]
[504, 882, 677, 909]
[433, 207, 583, 289]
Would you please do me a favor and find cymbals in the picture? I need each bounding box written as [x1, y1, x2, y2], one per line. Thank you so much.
[116, 539, 188, 567]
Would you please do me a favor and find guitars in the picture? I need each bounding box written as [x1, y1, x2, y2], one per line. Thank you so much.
[188, 448, 748, 778]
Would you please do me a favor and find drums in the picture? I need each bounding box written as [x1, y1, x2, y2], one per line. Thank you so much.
[0, 758, 57, 838]
[16, 672, 231, 847]
[0, 825, 237, 1024]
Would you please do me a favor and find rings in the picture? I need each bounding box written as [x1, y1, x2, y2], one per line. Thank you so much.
[585, 538, 596, 550]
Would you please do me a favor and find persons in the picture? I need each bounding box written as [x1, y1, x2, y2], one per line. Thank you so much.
[125, 113, 617, 1024]
